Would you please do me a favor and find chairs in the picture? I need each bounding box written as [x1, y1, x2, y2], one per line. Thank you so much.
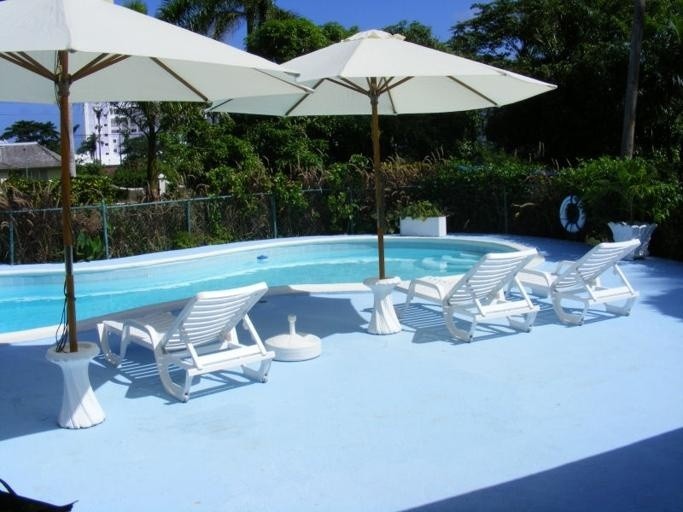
[404, 240, 640, 344]
[97, 281, 275, 401]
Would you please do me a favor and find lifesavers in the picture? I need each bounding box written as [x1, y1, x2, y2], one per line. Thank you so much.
[559, 195, 587, 233]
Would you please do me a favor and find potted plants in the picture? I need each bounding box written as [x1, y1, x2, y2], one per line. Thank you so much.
[397, 198, 448, 239]
[581, 158, 681, 258]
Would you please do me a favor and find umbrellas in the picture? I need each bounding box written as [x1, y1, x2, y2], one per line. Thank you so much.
[204, 26, 560, 279]
[0, 0, 313, 354]
